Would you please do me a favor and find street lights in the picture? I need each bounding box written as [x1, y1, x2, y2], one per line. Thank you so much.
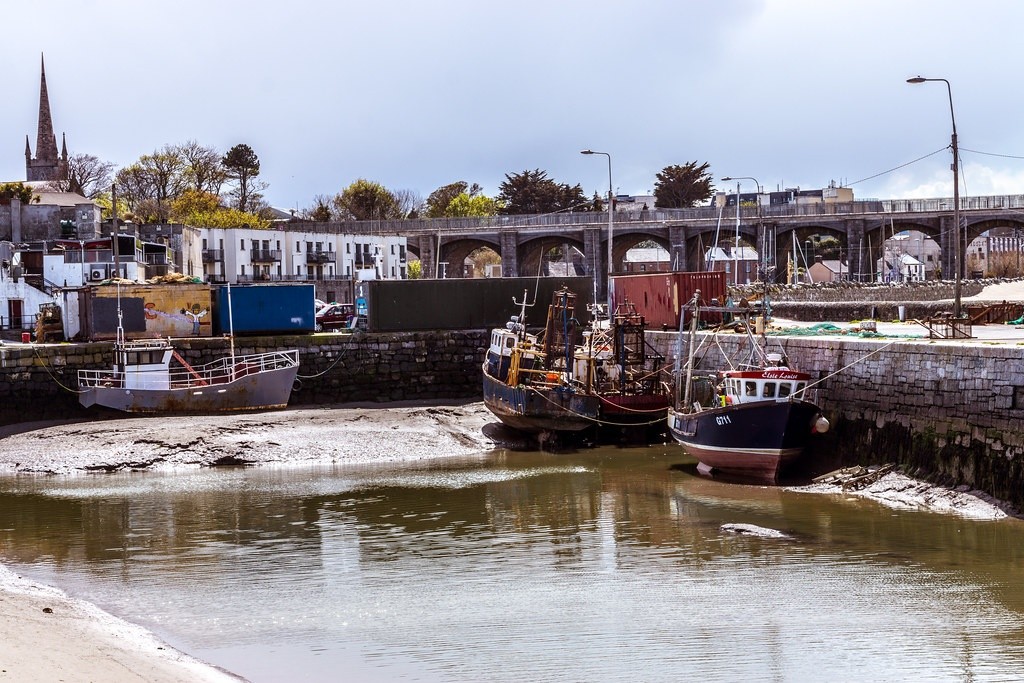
[906, 78, 960, 317]
[721, 177, 761, 281]
[581, 150, 613, 306]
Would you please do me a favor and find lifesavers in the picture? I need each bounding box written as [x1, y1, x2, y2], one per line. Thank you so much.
[292, 378, 303, 392]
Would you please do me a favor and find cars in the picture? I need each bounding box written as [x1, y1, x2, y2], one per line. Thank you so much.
[315, 299, 335, 315]
[316, 304, 354, 333]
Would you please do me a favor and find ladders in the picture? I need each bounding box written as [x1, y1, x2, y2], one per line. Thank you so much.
[153, 332, 209, 386]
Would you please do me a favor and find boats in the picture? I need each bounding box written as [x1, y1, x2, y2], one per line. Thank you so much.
[77, 283, 300, 413]
[482, 284, 668, 434]
[667, 279, 825, 481]
